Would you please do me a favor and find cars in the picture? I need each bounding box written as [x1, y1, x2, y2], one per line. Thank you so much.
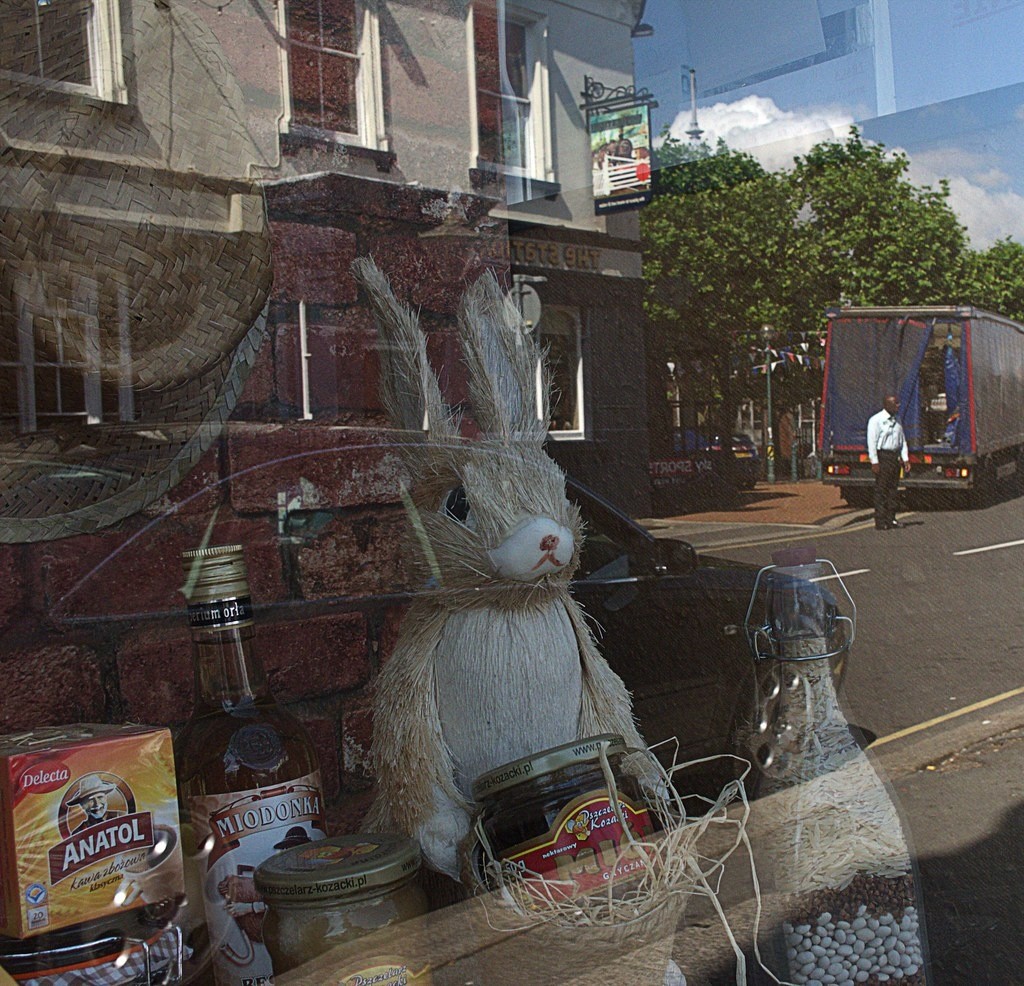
[1, 419, 935, 986]
[665, 425, 760, 488]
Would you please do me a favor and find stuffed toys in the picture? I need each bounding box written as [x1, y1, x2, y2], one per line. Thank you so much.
[344, 252, 697, 986]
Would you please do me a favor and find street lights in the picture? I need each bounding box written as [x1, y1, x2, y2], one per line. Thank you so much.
[759, 325, 780, 485]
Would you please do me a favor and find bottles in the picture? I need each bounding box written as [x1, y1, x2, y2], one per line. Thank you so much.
[742, 548, 937, 985]
[257, 838, 437, 986]
[458, 728, 692, 967]
[181, 553, 331, 986]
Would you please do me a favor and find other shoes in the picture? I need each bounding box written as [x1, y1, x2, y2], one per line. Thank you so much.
[876, 520, 905, 530]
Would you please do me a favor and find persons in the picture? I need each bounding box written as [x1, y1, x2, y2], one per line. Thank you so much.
[592, 125, 653, 205]
[867, 392, 911, 531]
[64, 775, 128, 836]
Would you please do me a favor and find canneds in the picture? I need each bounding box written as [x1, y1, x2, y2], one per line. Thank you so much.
[254, 733, 655, 985]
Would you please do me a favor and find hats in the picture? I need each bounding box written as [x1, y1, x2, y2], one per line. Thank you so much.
[64, 774, 116, 806]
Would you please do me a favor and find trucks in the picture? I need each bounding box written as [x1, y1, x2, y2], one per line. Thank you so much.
[818, 305, 1022, 511]
